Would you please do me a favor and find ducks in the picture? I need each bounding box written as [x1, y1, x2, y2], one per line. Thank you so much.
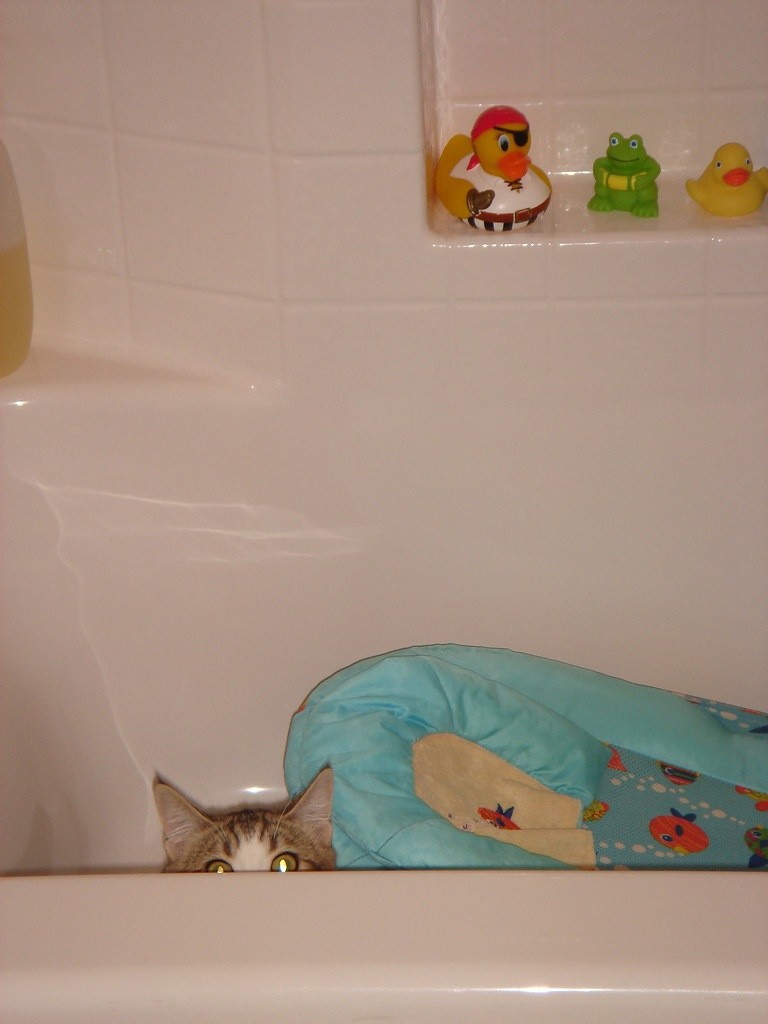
[685, 142, 768, 218]
[435, 105, 552, 231]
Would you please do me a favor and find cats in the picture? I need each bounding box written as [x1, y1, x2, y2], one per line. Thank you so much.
[149, 764, 337, 873]
